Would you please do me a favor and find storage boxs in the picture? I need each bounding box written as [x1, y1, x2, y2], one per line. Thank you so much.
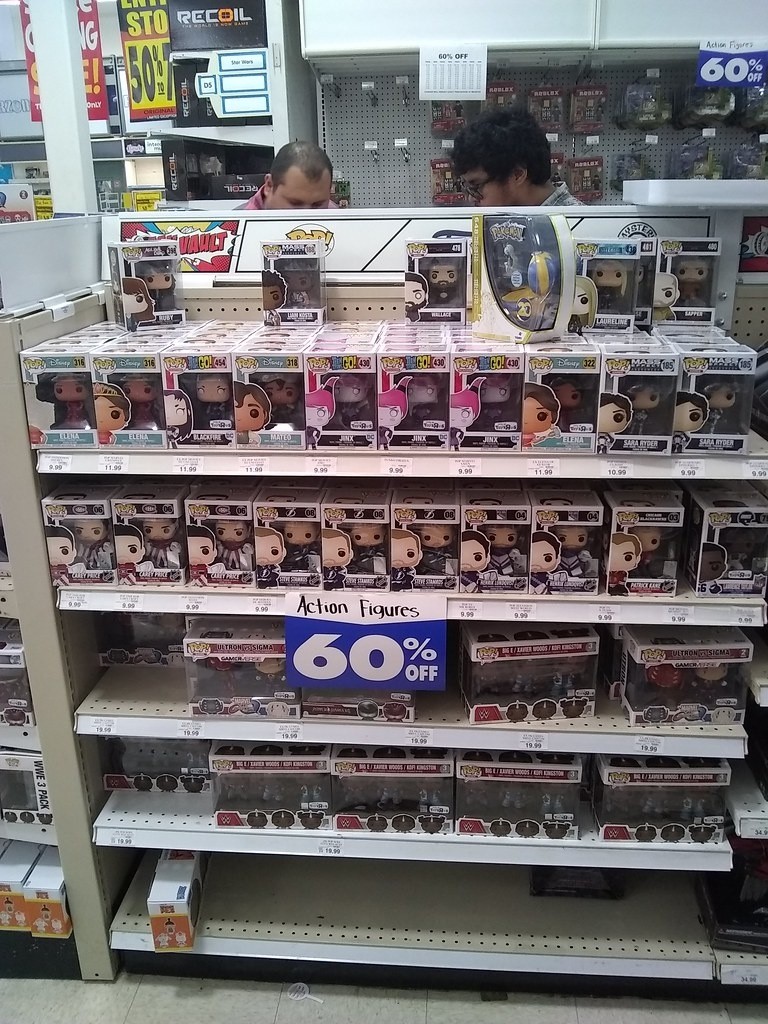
[0, 624, 75, 940]
[146, 851, 207, 951]
[18, 238, 767, 842]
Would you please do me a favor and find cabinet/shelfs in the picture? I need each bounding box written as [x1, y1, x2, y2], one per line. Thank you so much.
[0, 721, 100, 980]
[707, 639, 768, 987]
[0, 211, 767, 989]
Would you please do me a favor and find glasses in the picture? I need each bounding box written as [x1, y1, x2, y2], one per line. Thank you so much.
[462, 178, 496, 202]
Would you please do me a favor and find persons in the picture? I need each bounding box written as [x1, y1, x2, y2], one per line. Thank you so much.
[585, 259, 629, 316]
[70, 517, 756, 587]
[136, 260, 176, 311]
[424, 257, 461, 308]
[278, 258, 314, 310]
[1, 617, 744, 818]
[447, 105, 586, 207]
[670, 254, 711, 308]
[234, 141, 341, 211]
[50, 372, 744, 443]
